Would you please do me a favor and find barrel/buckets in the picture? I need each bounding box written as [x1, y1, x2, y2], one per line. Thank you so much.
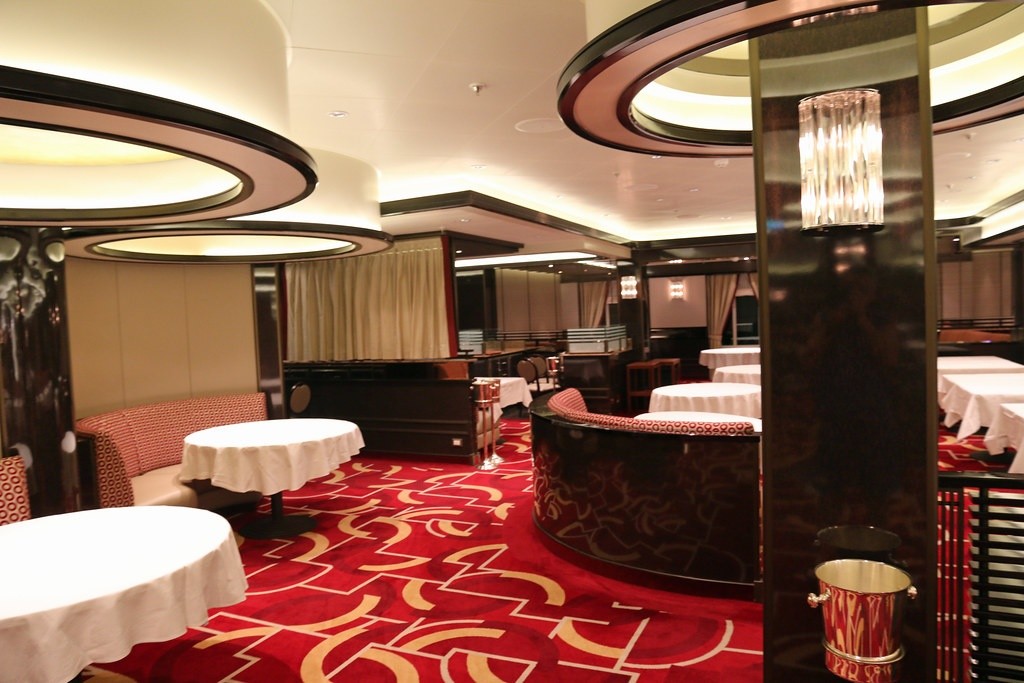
[807, 558, 918, 683]
[471, 379, 501, 408]
[547, 356, 560, 373]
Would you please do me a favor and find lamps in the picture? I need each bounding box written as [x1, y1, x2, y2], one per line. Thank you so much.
[620, 275, 638, 299]
[794, 89, 885, 232]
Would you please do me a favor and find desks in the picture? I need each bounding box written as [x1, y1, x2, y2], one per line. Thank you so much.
[981, 402, 1024, 474]
[938, 373, 1024, 461]
[179, 417, 366, 537]
[472, 376, 531, 445]
[634, 410, 762, 477]
[698, 348, 761, 368]
[936, 355, 1024, 394]
[0, 504, 249, 683]
[648, 381, 761, 417]
[712, 364, 760, 383]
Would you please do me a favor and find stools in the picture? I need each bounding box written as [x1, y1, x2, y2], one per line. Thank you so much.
[626, 361, 659, 412]
[652, 358, 681, 386]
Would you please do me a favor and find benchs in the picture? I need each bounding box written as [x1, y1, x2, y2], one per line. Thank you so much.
[73, 392, 267, 508]
[527, 386, 760, 602]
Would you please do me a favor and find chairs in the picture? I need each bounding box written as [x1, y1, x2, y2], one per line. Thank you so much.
[516, 357, 555, 420]
